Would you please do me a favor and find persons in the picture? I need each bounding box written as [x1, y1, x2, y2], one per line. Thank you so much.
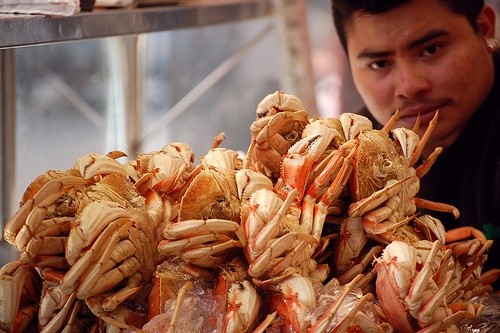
[331, 1, 500, 296]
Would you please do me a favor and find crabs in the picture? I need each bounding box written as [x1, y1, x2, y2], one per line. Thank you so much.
[1, 91, 500, 333]
